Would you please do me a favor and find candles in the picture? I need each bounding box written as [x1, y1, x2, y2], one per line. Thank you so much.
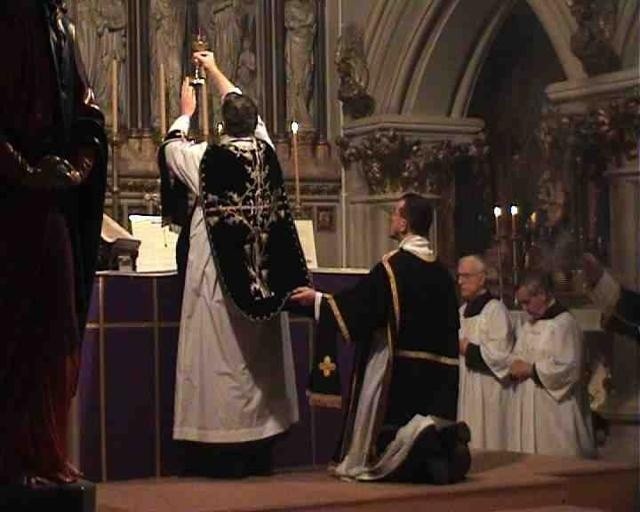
[112, 58, 118, 134]
[159, 63, 166, 135]
[203, 80, 209, 135]
[494, 207, 501, 234]
[511, 206, 518, 233]
[292, 121, 300, 203]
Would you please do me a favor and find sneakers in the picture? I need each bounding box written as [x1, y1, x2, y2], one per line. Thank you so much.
[442, 421, 474, 484]
[408, 425, 442, 481]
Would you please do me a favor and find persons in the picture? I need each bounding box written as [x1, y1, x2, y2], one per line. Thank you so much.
[506, 276, 598, 454]
[163, 50, 341, 476]
[581, 254, 638, 336]
[1, 0, 105, 490]
[452, 254, 513, 446]
[287, 192, 471, 486]
